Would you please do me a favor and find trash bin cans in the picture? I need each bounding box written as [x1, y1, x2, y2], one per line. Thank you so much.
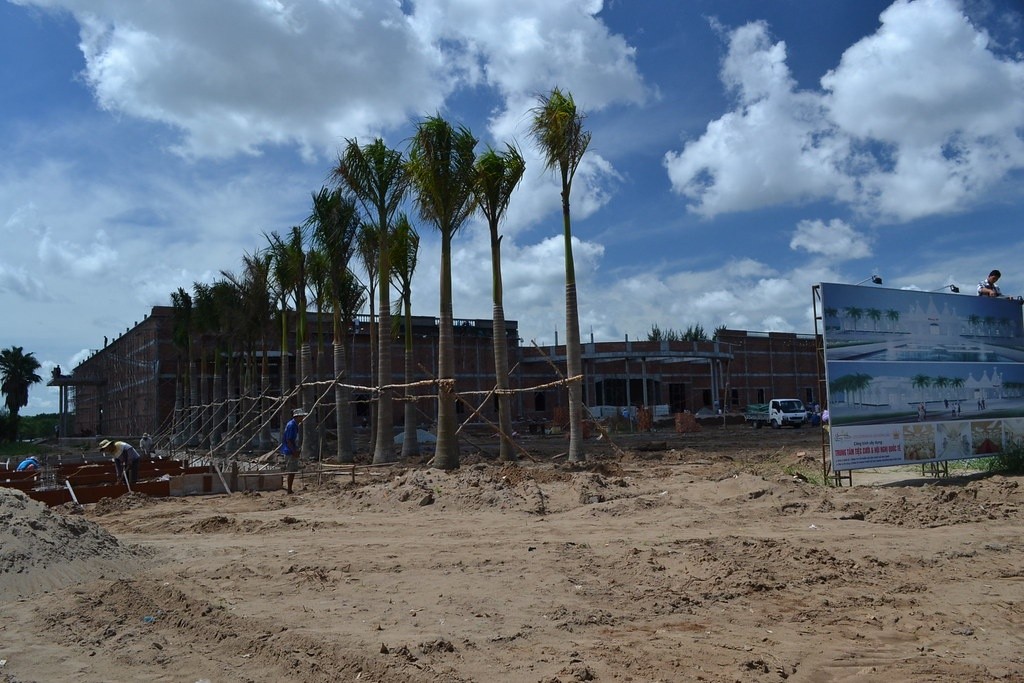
[811, 414, 818, 427]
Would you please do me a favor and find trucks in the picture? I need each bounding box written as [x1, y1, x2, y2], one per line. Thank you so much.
[743, 398, 807, 429]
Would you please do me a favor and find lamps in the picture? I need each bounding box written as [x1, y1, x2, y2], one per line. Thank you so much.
[856, 274, 882, 285]
[932, 285, 960, 293]
[1002, 295, 1024, 305]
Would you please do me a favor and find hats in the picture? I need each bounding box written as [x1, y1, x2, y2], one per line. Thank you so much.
[30, 455, 39, 462]
[292, 408, 308, 418]
[98, 439, 114, 452]
[142, 432, 148, 437]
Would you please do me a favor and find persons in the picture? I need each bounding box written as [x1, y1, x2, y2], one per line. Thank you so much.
[99, 439, 140, 484]
[944, 399, 961, 416]
[623, 408, 629, 417]
[808, 402, 820, 413]
[978, 270, 1013, 300]
[140, 433, 151, 454]
[281, 409, 308, 494]
[918, 404, 926, 421]
[17, 457, 39, 470]
[55, 423, 59, 438]
[714, 400, 720, 413]
[978, 397, 986, 411]
